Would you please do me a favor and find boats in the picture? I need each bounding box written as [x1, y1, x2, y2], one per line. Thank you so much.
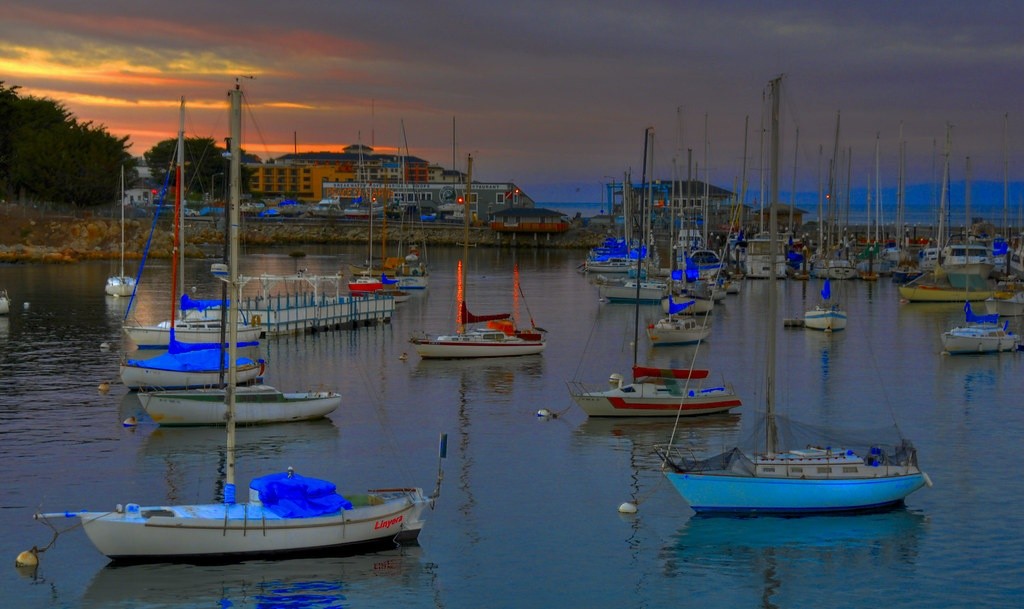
[0, 288, 13, 315]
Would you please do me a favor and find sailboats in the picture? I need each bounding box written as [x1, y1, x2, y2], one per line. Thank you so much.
[655, 74, 936, 516]
[27, 78, 450, 564]
[104, 66, 1024, 427]
